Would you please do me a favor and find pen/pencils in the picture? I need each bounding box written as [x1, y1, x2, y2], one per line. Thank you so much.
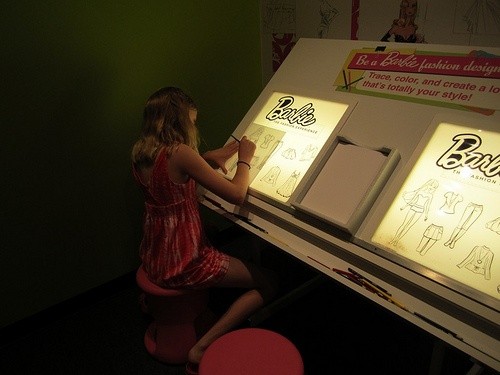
[333, 265, 464, 342]
[202, 194, 269, 234]
[307, 255, 332, 272]
[230, 134, 241, 146]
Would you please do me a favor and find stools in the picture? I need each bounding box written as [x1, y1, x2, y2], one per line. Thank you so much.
[199, 328, 304, 375]
[136, 264, 220, 363]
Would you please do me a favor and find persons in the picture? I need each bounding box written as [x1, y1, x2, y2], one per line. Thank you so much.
[132, 87, 277, 375]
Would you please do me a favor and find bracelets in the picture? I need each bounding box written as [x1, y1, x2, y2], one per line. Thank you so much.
[237, 161, 251, 170]
